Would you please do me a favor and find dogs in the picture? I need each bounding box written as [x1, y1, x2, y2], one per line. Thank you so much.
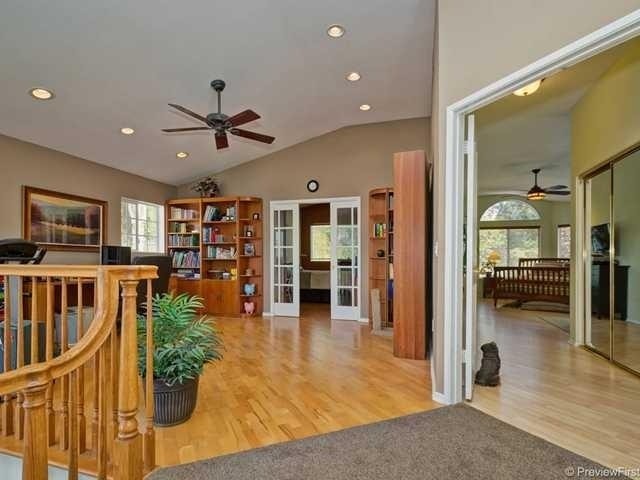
[474, 340, 502, 386]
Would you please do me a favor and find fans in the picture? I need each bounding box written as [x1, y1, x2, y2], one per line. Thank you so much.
[497, 168, 572, 201]
[160, 79, 277, 152]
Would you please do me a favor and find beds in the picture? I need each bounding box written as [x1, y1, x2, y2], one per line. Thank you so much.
[494, 257, 569, 309]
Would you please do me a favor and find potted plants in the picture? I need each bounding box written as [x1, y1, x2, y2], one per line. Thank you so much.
[136, 288, 228, 427]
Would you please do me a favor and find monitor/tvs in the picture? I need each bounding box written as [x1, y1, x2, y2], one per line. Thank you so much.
[590, 222, 616, 257]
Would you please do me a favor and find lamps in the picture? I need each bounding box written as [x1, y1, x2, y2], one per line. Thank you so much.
[526, 185, 545, 201]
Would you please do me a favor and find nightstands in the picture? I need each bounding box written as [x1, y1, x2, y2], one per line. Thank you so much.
[483, 277, 494, 298]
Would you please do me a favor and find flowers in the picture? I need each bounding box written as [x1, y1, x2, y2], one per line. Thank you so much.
[479, 252, 500, 274]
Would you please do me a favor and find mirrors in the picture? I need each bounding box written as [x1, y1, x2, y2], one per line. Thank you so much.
[589, 148, 639, 374]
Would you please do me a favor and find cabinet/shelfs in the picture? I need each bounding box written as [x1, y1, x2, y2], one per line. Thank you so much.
[164, 196, 264, 316]
[367, 187, 393, 328]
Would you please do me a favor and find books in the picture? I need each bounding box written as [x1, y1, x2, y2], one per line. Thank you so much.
[168, 206, 235, 281]
[373, 223, 386, 238]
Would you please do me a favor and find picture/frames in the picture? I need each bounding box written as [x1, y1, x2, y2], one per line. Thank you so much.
[20, 183, 110, 254]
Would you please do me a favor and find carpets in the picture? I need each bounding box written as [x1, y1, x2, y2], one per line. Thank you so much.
[143, 400, 633, 480]
[538, 315, 570, 334]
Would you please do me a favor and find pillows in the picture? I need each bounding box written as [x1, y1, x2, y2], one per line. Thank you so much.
[530, 261, 564, 277]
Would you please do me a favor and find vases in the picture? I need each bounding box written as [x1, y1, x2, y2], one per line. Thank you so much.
[486, 272, 493, 278]
